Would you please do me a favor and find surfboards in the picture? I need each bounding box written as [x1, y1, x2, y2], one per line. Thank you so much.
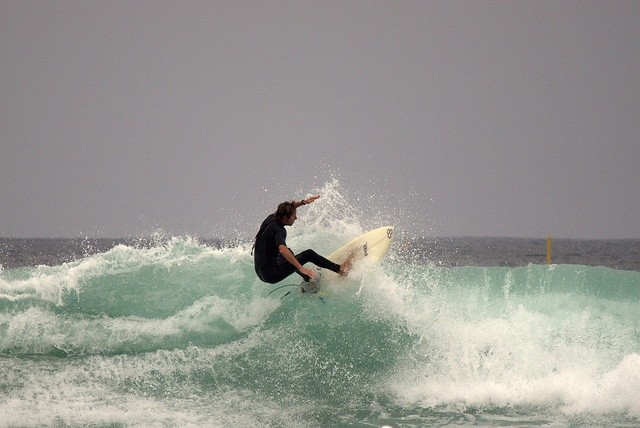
[278, 225, 395, 307]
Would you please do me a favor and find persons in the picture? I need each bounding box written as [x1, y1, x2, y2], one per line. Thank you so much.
[254, 194, 358, 285]
